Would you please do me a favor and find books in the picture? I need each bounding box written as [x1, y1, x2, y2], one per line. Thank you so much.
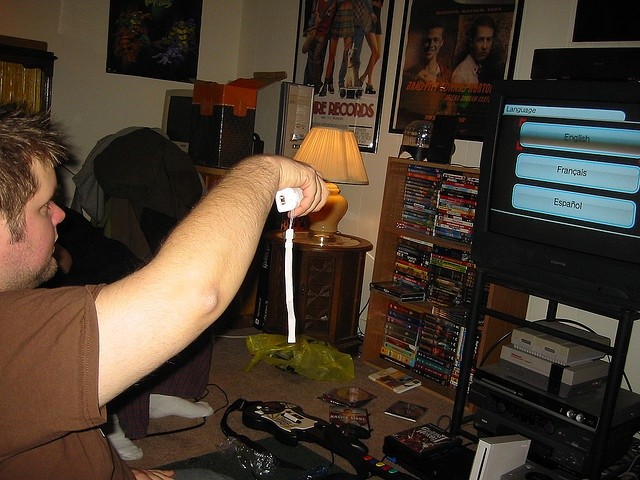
[368, 300, 483, 397]
[382, 422, 463, 472]
[329, 407, 373, 440]
[401, 164, 480, 245]
[369, 239, 491, 327]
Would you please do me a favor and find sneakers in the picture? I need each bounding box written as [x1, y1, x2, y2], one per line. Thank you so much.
[327, 80, 334, 94]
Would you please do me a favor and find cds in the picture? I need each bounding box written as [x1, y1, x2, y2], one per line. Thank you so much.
[337, 387, 369, 403]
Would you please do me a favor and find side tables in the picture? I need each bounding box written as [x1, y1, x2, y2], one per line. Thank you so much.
[263, 229, 372, 356]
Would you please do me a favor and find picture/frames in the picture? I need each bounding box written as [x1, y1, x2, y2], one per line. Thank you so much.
[389, 0, 524, 143]
[292, 1, 395, 154]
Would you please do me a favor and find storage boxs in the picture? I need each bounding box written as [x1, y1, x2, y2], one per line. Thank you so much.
[187, 70, 288, 169]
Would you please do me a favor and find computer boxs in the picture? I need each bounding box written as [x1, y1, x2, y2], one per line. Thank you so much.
[252, 82, 315, 159]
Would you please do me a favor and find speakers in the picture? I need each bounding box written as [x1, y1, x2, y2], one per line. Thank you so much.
[428, 116, 457, 163]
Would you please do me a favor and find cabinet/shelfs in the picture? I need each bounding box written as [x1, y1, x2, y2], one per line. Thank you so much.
[1, 45, 57, 136]
[362, 156, 530, 392]
[450, 267, 640, 480]
[191, 164, 262, 333]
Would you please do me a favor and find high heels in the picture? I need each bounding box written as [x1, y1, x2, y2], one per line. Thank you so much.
[365, 83, 376, 94]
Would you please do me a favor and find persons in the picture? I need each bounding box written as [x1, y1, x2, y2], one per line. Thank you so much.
[303, 0, 318, 85]
[312, 0, 334, 95]
[1, 99, 329, 480]
[396, 25, 453, 130]
[358, 0, 384, 94]
[338, 0, 364, 89]
[450, 15, 497, 134]
[319, 0, 354, 96]
[43, 196, 214, 465]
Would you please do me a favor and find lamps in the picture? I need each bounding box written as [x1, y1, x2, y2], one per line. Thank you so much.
[292, 126, 369, 241]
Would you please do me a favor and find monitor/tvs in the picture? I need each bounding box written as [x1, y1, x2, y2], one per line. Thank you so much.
[162, 89, 194, 153]
[471, 80, 639, 310]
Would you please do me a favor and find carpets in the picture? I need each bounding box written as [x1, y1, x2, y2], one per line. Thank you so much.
[148, 434, 359, 480]
